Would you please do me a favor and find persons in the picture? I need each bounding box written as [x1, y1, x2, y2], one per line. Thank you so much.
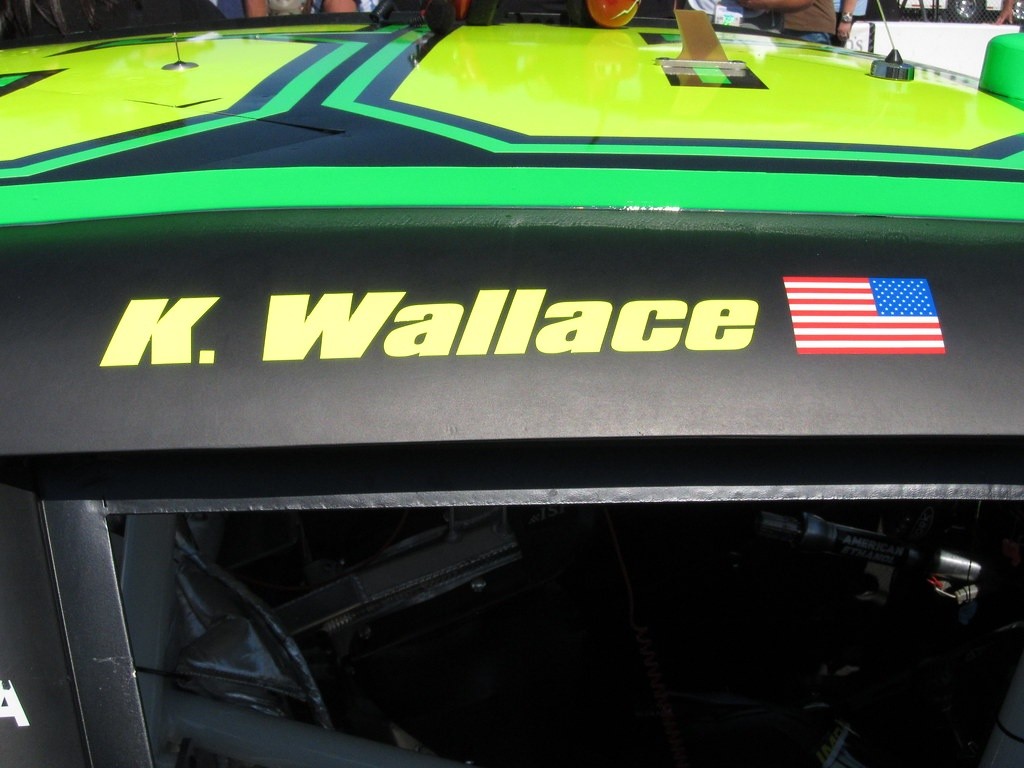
[684, 0, 857, 48]
[240, 0, 360, 18]
[993, 0, 1017, 26]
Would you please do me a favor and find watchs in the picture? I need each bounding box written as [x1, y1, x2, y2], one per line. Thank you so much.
[841, 15, 852, 22]
